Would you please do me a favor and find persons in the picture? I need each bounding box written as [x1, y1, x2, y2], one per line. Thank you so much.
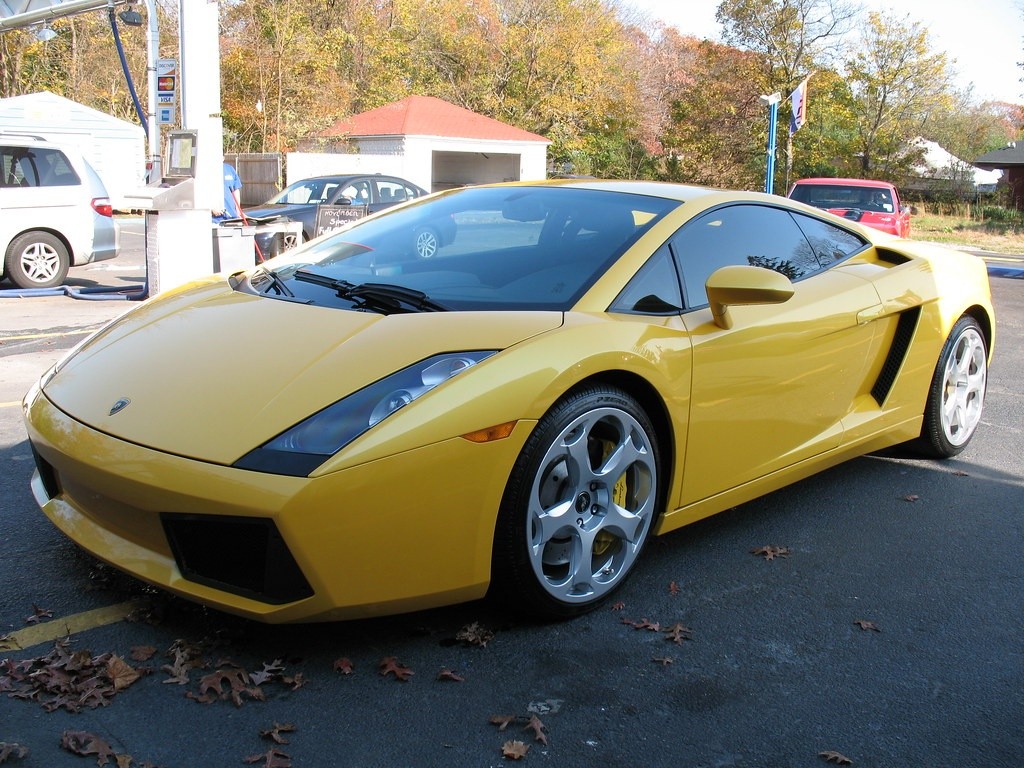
[852, 190, 878, 210]
[210, 148, 242, 224]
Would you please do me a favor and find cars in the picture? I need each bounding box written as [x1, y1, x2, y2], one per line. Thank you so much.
[221, 173, 458, 263]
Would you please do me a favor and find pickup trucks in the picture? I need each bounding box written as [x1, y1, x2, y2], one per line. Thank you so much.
[786, 177, 911, 239]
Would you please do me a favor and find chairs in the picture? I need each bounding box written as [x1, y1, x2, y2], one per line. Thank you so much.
[356, 188, 371, 206]
[328, 186, 343, 206]
[380, 188, 391, 205]
[392, 189, 407, 205]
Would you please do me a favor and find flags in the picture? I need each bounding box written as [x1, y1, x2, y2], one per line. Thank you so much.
[789, 80, 807, 138]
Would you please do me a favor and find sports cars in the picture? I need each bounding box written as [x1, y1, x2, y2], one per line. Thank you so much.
[21, 179, 998, 628]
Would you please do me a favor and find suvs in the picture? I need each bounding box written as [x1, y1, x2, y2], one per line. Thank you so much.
[0, 133, 122, 288]
